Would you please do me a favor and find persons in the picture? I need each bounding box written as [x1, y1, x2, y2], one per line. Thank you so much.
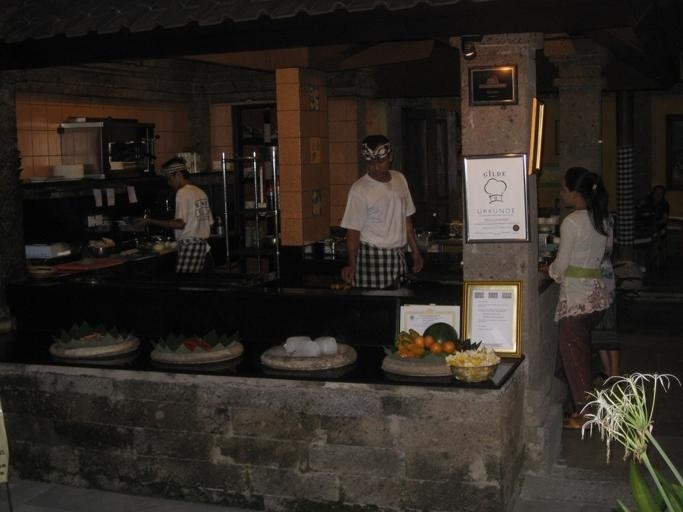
[133, 157, 215, 275]
[340, 135, 424, 288]
[540, 166, 620, 429]
[636, 185, 670, 231]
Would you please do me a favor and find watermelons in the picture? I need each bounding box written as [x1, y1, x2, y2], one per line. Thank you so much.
[422, 322, 457, 344]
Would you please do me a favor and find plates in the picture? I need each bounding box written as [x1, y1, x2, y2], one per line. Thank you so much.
[54, 164, 84, 181]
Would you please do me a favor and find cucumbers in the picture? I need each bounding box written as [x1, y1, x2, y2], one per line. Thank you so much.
[615, 459, 683, 512]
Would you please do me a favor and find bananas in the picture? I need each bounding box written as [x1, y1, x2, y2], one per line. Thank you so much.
[393, 329, 424, 358]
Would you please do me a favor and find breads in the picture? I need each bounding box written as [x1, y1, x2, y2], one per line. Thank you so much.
[284, 336, 312, 353]
[315, 336, 338, 355]
[293, 340, 320, 358]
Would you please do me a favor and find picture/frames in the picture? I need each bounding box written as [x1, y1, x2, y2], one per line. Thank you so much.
[462, 280, 524, 360]
[460, 154, 531, 244]
[467, 65, 517, 106]
[393, 296, 461, 345]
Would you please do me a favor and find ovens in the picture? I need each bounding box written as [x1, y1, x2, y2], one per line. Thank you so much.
[57, 117, 160, 180]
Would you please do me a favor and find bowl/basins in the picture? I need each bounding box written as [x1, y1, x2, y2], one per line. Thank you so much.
[450, 363, 499, 383]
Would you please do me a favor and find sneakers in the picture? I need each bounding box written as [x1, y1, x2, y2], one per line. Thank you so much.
[563, 411, 595, 429]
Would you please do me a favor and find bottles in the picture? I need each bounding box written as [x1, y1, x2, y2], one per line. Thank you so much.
[267, 182, 274, 211]
